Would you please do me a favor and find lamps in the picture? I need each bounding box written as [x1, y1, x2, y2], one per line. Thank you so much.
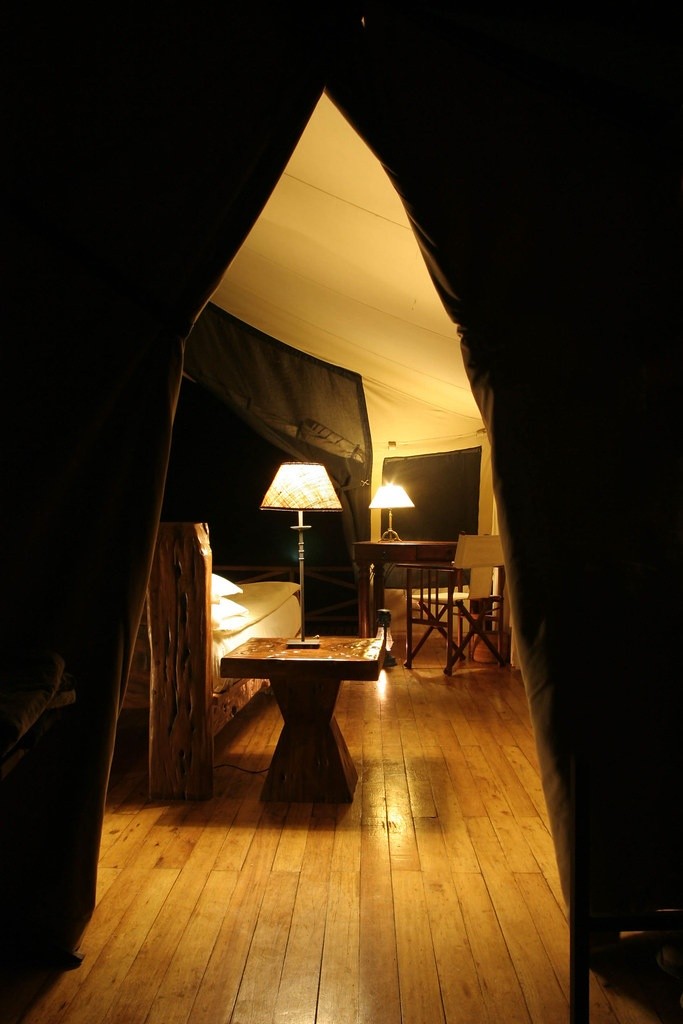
[367, 485, 415, 543]
[259, 460, 344, 648]
[375, 608, 397, 668]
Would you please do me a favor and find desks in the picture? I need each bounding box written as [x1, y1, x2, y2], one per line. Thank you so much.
[220, 637, 386, 806]
[351, 538, 466, 638]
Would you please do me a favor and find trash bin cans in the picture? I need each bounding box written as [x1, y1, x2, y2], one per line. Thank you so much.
[471, 629, 500, 664]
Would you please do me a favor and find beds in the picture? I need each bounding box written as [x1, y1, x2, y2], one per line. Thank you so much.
[122, 520, 302, 804]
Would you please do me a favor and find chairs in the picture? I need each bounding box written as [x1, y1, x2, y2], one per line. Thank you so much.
[567, 741, 683, 1024]
[395, 529, 507, 677]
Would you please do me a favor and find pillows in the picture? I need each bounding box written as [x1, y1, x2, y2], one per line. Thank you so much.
[210, 597, 254, 631]
[210, 574, 244, 602]
